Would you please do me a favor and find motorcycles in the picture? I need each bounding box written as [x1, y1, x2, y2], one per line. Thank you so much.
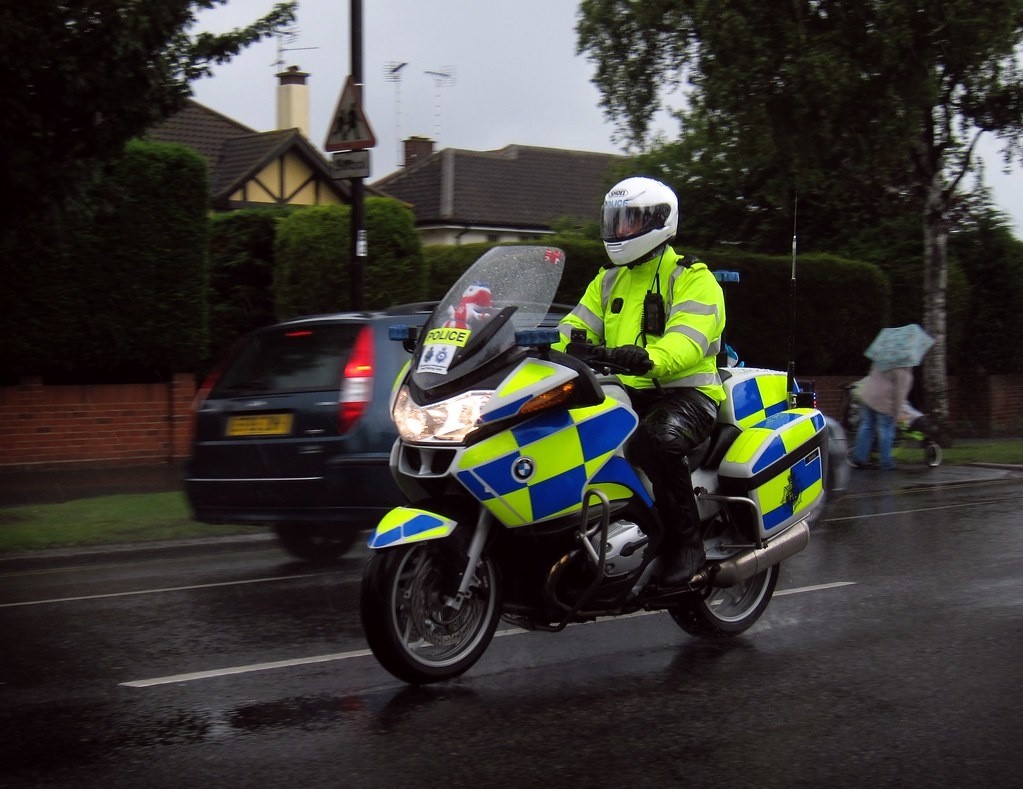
[360, 244, 832, 687]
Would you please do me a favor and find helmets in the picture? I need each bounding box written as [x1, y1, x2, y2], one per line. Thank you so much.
[600, 176, 679, 265]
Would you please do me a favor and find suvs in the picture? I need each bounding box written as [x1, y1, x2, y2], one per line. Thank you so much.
[183, 301, 573, 564]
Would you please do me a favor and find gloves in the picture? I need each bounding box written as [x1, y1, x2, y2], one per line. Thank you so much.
[610, 345, 649, 376]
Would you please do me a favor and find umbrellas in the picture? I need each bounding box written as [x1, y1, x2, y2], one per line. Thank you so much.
[864, 324, 936, 372]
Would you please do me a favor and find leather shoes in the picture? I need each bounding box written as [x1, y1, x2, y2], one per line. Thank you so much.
[658, 540, 706, 587]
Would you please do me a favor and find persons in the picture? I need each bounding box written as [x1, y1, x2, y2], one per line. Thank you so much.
[847, 366, 913, 470]
[504, 174, 731, 629]
